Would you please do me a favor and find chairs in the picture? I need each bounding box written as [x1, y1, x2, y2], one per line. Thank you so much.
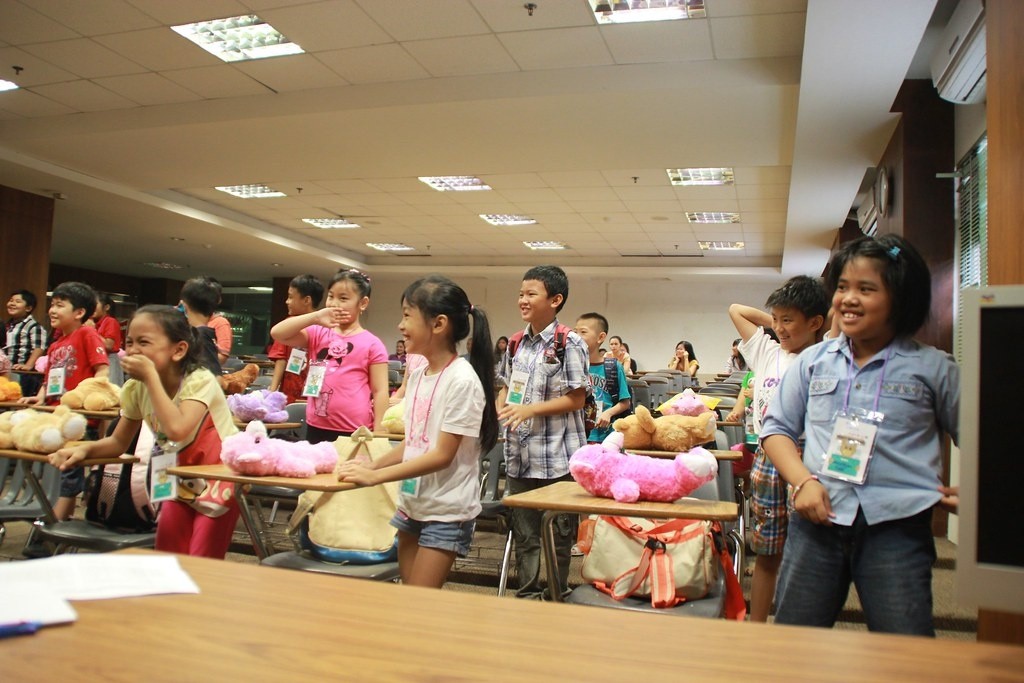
[0, 345, 753, 618]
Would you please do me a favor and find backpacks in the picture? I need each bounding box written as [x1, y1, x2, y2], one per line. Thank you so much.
[285, 425, 403, 566]
[80, 409, 162, 534]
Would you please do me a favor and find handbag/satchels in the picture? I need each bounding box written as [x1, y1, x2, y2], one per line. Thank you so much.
[578, 514, 746, 621]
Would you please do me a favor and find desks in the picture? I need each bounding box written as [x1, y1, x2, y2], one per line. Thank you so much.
[665, 390, 740, 397]
[371, 432, 404, 440]
[165, 463, 359, 559]
[624, 447, 744, 459]
[713, 420, 745, 427]
[0, 401, 31, 408]
[498, 480, 739, 596]
[0, 548, 1024, 683]
[31, 405, 120, 421]
[1, 442, 140, 523]
[236, 421, 304, 430]
[11, 370, 45, 374]
[713, 405, 735, 409]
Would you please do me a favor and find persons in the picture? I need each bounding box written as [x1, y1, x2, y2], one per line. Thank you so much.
[46, 304, 251, 561]
[5, 264, 845, 622]
[339, 276, 500, 591]
[759, 234, 963, 637]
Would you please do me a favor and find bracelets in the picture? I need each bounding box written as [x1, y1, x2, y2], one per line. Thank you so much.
[791, 475, 822, 512]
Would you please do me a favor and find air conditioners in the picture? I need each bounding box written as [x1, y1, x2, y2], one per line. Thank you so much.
[930, 0, 988, 104]
[856, 187, 877, 236]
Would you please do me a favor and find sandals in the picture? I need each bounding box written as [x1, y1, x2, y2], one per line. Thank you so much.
[571, 540, 585, 555]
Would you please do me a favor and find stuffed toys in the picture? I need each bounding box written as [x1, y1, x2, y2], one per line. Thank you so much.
[35, 355, 48, 372]
[0, 375, 22, 402]
[0, 404, 87, 453]
[61, 376, 121, 410]
[221, 419, 338, 478]
[216, 362, 260, 394]
[570, 432, 719, 503]
[382, 397, 406, 433]
[612, 405, 717, 453]
[669, 387, 719, 420]
[0, 348, 11, 370]
[227, 389, 289, 422]
